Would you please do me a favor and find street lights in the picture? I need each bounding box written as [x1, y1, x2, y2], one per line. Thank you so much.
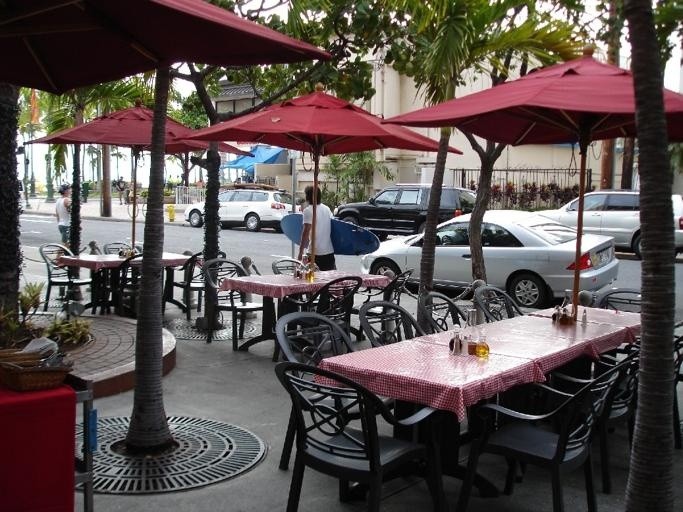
[29, 125, 36, 197]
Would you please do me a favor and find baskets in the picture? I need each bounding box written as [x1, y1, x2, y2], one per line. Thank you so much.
[0, 348, 73, 391]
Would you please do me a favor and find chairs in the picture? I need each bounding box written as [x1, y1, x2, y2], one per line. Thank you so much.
[275, 363, 444, 511]
[274, 309, 407, 500]
[37, 241, 527, 346]
[547, 287, 682, 494]
[454, 359, 637, 511]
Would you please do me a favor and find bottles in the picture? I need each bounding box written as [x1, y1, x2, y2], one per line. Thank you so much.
[448, 309, 489, 357]
[117, 246, 136, 259]
[552, 289, 587, 327]
[293, 253, 313, 282]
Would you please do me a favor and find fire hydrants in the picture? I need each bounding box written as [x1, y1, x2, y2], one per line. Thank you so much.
[167, 205, 176, 222]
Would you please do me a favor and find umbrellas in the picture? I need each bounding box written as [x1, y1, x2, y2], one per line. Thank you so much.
[22, 98, 254, 257]
[378, 46, 682, 322]
[171, 81, 463, 280]
[0, 1, 333, 96]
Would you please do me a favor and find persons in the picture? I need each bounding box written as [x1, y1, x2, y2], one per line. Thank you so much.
[53, 184, 72, 246]
[296, 185, 335, 271]
[114, 176, 134, 205]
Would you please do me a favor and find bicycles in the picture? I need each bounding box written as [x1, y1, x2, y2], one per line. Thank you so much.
[124, 193, 148, 218]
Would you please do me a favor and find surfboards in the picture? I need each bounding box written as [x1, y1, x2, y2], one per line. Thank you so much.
[278, 213, 380, 255]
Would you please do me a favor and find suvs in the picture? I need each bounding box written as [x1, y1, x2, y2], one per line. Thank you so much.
[333, 183, 477, 242]
[184, 189, 302, 232]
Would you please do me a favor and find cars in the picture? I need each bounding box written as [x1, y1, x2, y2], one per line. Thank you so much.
[531, 189, 682, 261]
[359, 210, 619, 309]
